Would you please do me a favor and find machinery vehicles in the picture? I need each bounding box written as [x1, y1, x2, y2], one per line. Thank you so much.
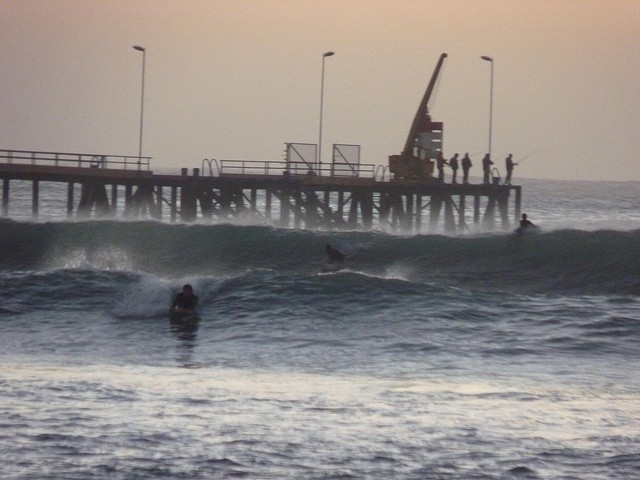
[388, 52, 447, 180]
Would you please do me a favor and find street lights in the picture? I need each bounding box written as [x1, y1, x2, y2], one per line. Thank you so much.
[480, 56, 493, 154]
[133, 46, 145, 170]
[318, 51, 334, 175]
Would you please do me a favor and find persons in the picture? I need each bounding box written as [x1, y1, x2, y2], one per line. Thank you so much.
[451, 153, 458, 184]
[518, 211, 538, 232]
[462, 152, 472, 184]
[483, 153, 495, 183]
[504, 153, 518, 185]
[171, 284, 198, 313]
[323, 241, 346, 266]
[436, 150, 448, 183]
